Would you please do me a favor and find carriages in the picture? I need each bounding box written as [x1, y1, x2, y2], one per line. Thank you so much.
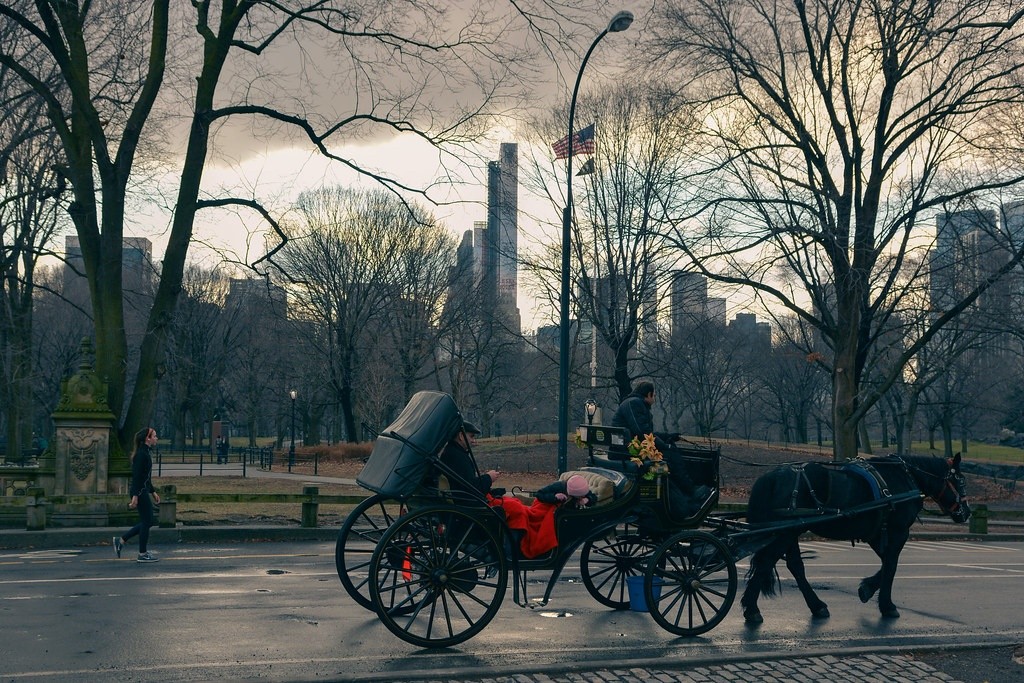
[335, 391, 971, 648]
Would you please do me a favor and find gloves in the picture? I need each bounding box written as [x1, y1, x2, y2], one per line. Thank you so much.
[555, 493, 567, 501]
[577, 498, 588, 505]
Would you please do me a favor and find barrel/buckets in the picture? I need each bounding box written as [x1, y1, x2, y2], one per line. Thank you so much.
[629, 575, 664, 612]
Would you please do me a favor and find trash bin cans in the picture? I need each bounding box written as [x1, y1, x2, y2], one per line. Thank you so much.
[969, 503, 988, 533]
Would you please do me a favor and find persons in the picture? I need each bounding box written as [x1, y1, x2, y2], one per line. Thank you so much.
[112, 427, 161, 563]
[607, 381, 716, 521]
[215, 435, 222, 464]
[221, 436, 229, 464]
[536, 475, 598, 508]
[439, 420, 506, 508]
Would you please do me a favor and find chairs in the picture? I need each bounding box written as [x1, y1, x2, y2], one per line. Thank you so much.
[579, 424, 720, 529]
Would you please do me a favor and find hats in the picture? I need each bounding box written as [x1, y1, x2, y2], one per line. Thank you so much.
[567, 475, 589, 496]
[462, 420, 481, 434]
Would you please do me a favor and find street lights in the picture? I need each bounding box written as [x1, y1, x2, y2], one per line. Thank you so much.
[584, 399, 597, 455]
[290, 389, 298, 465]
[558, 10, 634, 477]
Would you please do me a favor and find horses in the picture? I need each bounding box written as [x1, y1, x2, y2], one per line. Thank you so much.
[745, 452, 972, 626]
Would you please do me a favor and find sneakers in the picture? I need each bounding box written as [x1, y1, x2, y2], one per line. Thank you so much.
[113, 537, 123, 558]
[137, 552, 159, 562]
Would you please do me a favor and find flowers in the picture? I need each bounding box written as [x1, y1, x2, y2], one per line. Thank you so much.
[628, 433, 663, 480]
[573, 427, 588, 451]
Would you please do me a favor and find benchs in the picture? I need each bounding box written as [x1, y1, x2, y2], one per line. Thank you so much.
[554, 467, 640, 552]
[355, 390, 506, 571]
